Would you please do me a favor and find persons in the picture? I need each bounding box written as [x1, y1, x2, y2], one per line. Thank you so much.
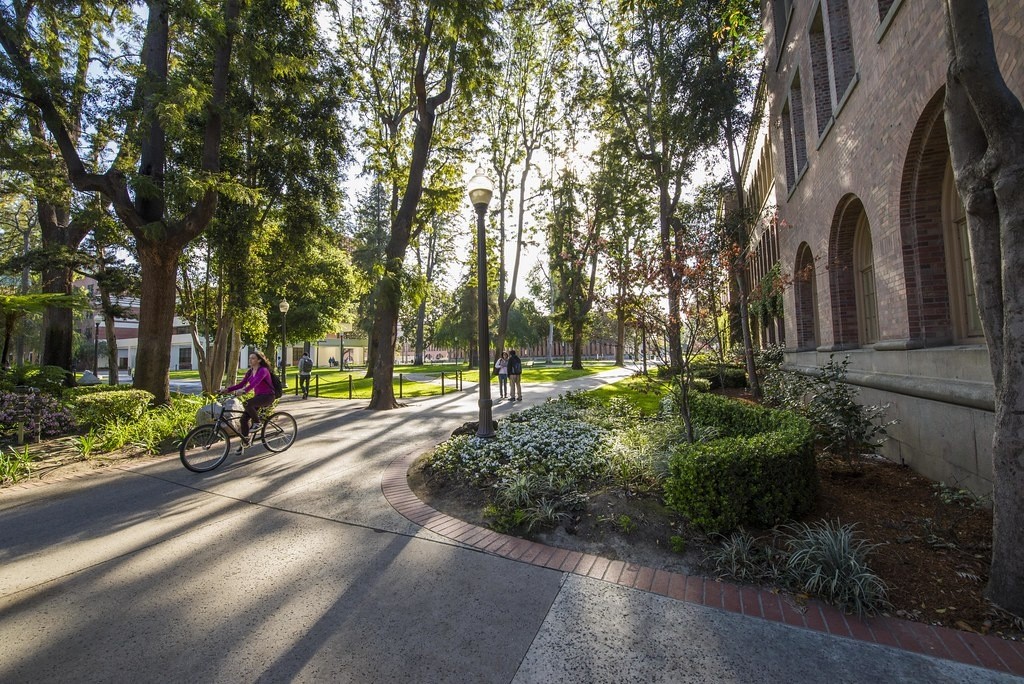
[507, 350, 522, 401]
[495, 352, 509, 399]
[277, 357, 281, 376]
[214, 351, 275, 455]
[298, 352, 311, 400]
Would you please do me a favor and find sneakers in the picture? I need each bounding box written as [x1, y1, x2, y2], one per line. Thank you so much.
[235, 441, 250, 455]
[249, 422, 264, 433]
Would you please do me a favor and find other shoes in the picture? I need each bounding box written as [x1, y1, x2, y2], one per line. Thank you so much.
[302, 393, 307, 398]
[508, 398, 515, 401]
[517, 398, 522, 400]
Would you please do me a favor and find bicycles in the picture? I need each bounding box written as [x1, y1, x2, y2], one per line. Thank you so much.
[179, 389, 297, 474]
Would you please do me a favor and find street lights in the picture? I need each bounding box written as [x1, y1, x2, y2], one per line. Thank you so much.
[465, 161, 497, 440]
[340, 330, 344, 372]
[279, 296, 288, 389]
[93, 312, 102, 378]
[316, 338, 319, 370]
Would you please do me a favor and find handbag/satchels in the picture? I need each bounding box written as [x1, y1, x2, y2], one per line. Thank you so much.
[493, 359, 501, 375]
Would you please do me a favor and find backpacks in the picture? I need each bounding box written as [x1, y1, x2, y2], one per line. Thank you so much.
[248, 366, 282, 399]
[512, 357, 521, 374]
[303, 357, 312, 373]
